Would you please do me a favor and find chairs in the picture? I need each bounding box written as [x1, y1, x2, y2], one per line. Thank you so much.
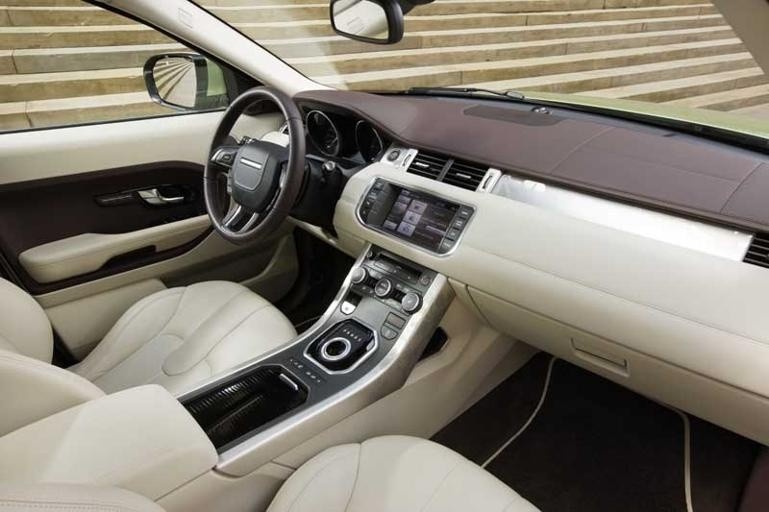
[0, 276, 298, 439]
[0, 435, 543, 512]
